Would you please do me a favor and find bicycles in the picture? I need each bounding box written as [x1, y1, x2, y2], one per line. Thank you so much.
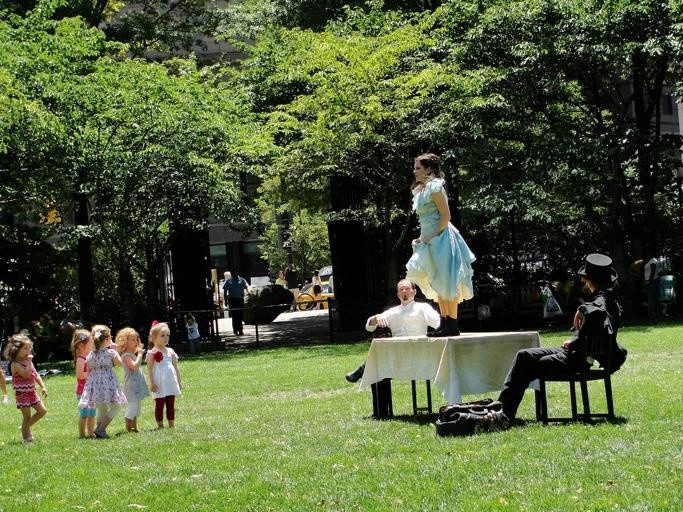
[289, 292, 314, 311]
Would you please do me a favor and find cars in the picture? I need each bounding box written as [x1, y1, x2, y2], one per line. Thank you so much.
[475, 249, 588, 291]
[297, 281, 335, 309]
[299, 283, 314, 295]
[217, 281, 229, 309]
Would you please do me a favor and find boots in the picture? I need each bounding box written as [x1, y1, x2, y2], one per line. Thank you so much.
[426, 315, 460, 336]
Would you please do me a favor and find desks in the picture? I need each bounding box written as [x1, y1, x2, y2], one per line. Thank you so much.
[356, 327, 548, 431]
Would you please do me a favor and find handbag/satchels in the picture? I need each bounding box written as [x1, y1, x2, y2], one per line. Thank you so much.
[436, 398, 509, 436]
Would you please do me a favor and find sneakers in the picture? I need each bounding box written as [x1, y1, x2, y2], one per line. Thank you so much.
[93, 426, 108, 438]
[345, 366, 364, 382]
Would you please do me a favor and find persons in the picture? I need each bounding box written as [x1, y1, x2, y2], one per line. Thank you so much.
[4, 334, 48, 442]
[346, 279, 441, 420]
[78, 324, 128, 439]
[71, 329, 96, 439]
[0, 358, 8, 403]
[115, 327, 150, 432]
[404, 153, 478, 337]
[184, 268, 335, 357]
[0, 306, 84, 361]
[644, 248, 659, 321]
[145, 320, 182, 428]
[496, 253, 626, 423]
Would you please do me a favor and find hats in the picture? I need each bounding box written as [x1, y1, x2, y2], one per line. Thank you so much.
[577, 253, 619, 284]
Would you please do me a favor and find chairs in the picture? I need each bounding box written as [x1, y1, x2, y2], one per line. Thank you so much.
[518, 322, 620, 423]
[368, 321, 435, 420]
